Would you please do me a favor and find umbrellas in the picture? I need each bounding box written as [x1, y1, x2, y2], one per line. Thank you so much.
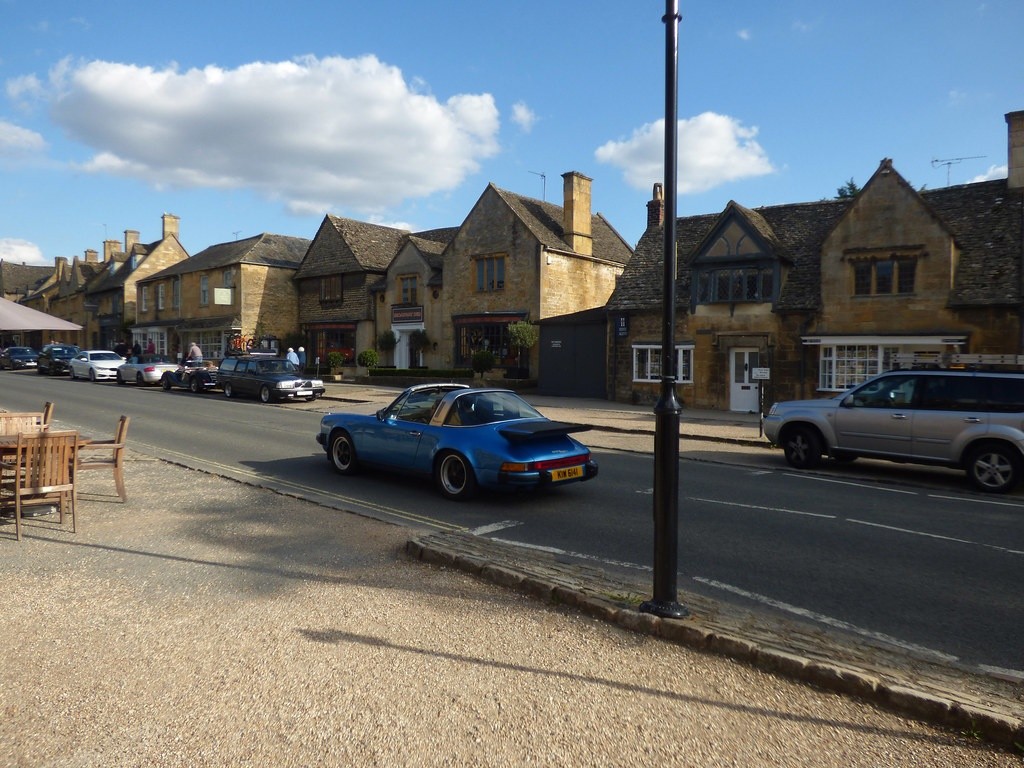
[0, 297, 84, 330]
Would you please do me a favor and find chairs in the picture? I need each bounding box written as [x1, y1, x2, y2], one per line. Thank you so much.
[0, 413, 44, 470]
[69, 414, 130, 502]
[1, 430, 78, 540]
[26, 401, 54, 432]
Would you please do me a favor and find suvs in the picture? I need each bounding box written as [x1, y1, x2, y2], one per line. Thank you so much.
[760, 353, 1024, 494]
[216, 345, 327, 402]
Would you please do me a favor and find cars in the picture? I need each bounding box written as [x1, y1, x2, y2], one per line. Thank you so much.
[160, 360, 221, 393]
[36, 344, 82, 375]
[0, 347, 40, 369]
[69, 350, 127, 381]
[116, 353, 181, 386]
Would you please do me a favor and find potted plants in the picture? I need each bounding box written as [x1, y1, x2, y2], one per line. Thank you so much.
[327, 351, 344, 375]
[356, 349, 381, 376]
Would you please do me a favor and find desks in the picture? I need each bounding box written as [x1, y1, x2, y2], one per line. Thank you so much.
[0, 434, 93, 514]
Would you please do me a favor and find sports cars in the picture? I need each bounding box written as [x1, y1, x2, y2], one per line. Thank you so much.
[316, 383, 599, 502]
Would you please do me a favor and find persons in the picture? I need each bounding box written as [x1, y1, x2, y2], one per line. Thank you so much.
[286, 347, 305, 373]
[132, 340, 141, 364]
[116, 341, 131, 360]
[145, 339, 155, 362]
[74, 343, 80, 350]
[458, 395, 474, 420]
[186, 343, 203, 367]
[423, 400, 440, 423]
[206, 361, 217, 371]
